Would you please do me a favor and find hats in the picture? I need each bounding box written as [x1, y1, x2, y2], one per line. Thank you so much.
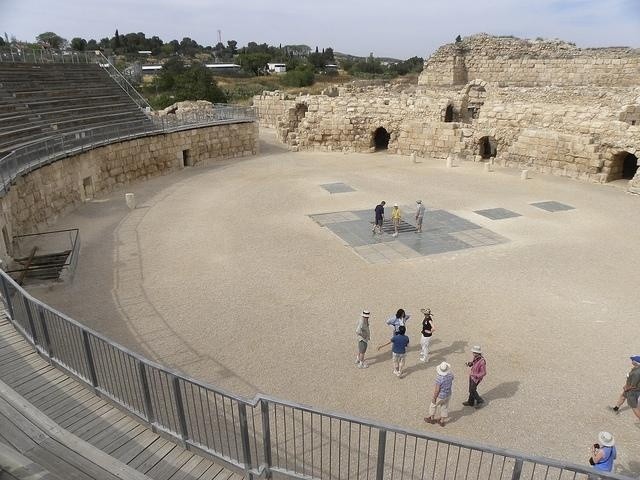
[630, 355, 640, 363]
[436, 362, 451, 376]
[361, 310, 370, 318]
[598, 432, 615, 447]
[470, 346, 482, 354]
[420, 308, 433, 316]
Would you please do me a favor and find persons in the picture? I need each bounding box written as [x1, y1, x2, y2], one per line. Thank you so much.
[391, 203, 401, 237]
[589, 431, 617, 480]
[414, 199, 425, 233]
[371, 200, 386, 234]
[462, 345, 487, 409]
[605, 355, 639, 429]
[424, 361, 453, 427]
[378, 326, 410, 377]
[387, 309, 411, 339]
[355, 310, 370, 369]
[419, 305, 436, 362]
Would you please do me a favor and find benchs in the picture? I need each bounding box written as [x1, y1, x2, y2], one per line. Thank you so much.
[0, 376, 152, 478]
[1, 55, 165, 175]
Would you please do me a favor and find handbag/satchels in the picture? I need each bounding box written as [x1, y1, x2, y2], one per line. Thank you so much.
[589, 457, 595, 465]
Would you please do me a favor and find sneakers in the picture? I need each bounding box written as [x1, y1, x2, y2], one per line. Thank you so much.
[463, 402, 470, 406]
[355, 350, 430, 377]
[438, 419, 444, 427]
[474, 401, 484, 408]
[424, 418, 436, 424]
